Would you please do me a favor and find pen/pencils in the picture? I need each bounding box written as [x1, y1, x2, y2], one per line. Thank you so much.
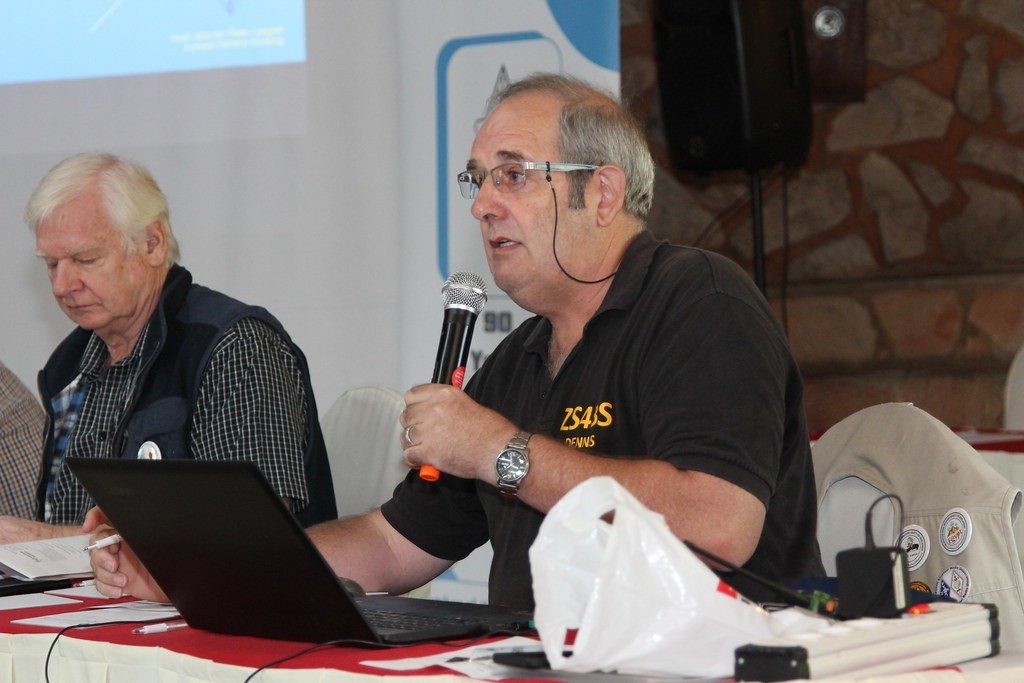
[83, 533, 122, 552]
[132, 621, 188, 635]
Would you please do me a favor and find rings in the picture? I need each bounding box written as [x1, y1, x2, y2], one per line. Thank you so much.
[405, 424, 415, 445]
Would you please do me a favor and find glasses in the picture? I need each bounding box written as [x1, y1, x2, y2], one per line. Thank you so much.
[456, 162, 599, 200]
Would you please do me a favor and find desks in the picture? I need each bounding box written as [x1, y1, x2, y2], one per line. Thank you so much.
[0, 579, 1024, 683]
[807, 427, 1024, 561]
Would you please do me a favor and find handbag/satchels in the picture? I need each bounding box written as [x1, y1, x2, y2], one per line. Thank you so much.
[529, 475, 826, 680]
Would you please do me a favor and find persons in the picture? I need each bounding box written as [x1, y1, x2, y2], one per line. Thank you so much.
[78, 72, 822, 604]
[0, 152, 340, 545]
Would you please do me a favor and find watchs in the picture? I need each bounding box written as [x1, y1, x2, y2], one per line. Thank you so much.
[494, 428, 536, 494]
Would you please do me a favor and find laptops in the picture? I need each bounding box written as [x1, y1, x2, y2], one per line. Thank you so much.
[65, 455, 536, 649]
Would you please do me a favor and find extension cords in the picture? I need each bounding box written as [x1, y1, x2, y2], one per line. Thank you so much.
[734, 601, 1002, 683]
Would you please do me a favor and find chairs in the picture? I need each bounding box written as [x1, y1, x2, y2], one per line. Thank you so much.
[318, 383, 432, 597]
[812, 401, 1024, 652]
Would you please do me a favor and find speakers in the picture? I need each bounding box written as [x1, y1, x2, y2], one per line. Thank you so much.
[652, 0, 813, 179]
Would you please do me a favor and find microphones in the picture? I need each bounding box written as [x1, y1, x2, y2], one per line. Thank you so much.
[418, 270, 487, 480]
